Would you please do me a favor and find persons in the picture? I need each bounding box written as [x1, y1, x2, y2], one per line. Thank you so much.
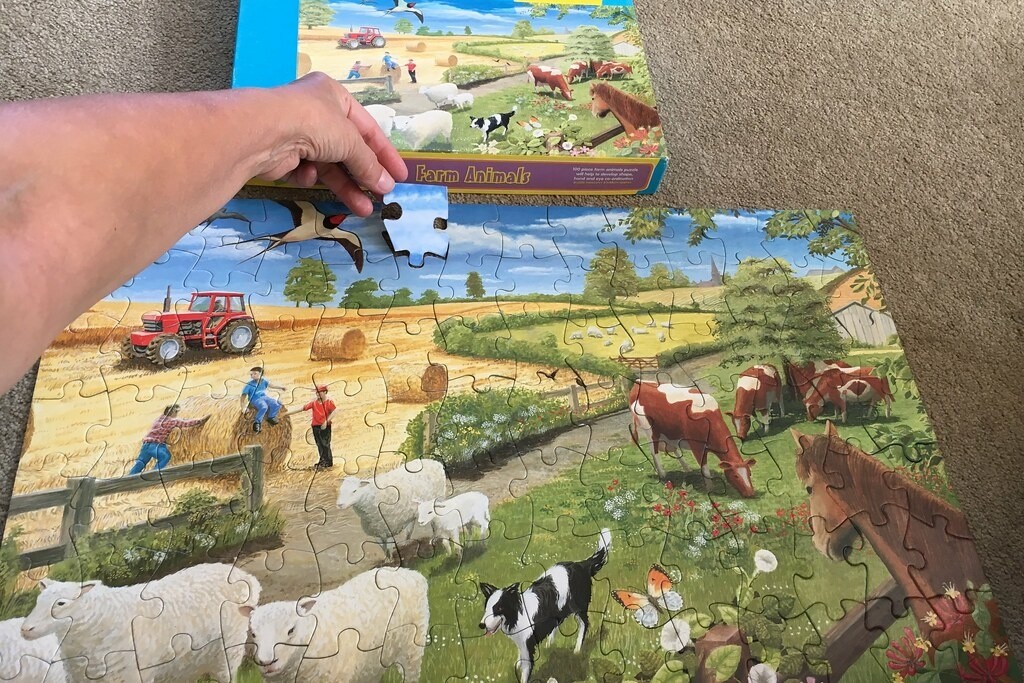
[0, 72, 409, 403]
